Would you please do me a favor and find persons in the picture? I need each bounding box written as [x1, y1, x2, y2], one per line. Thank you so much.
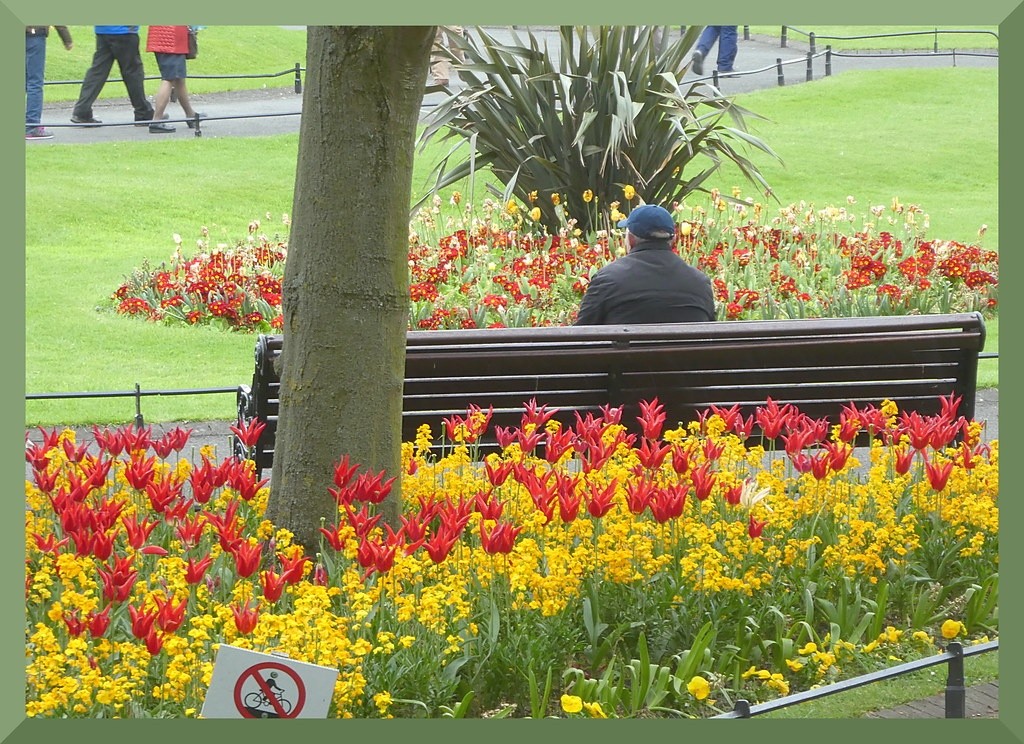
[70, 25, 169, 127]
[692, 26, 741, 78]
[430, 25, 466, 87]
[146, 26, 208, 133]
[572, 205, 717, 326]
[25, 26, 74, 140]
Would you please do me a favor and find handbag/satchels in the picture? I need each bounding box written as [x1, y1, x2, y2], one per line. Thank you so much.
[186, 30, 198, 59]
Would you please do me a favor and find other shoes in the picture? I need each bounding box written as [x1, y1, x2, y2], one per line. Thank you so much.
[717, 71, 740, 78]
[134, 114, 169, 127]
[149, 123, 176, 134]
[692, 50, 704, 76]
[187, 112, 207, 128]
[70, 115, 102, 127]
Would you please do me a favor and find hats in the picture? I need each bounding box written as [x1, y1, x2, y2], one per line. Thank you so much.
[617, 204, 676, 240]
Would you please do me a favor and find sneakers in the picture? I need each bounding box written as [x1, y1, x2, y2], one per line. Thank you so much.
[25, 126, 54, 140]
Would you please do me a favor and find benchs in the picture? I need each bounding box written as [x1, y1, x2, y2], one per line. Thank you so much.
[231, 311, 986, 489]
